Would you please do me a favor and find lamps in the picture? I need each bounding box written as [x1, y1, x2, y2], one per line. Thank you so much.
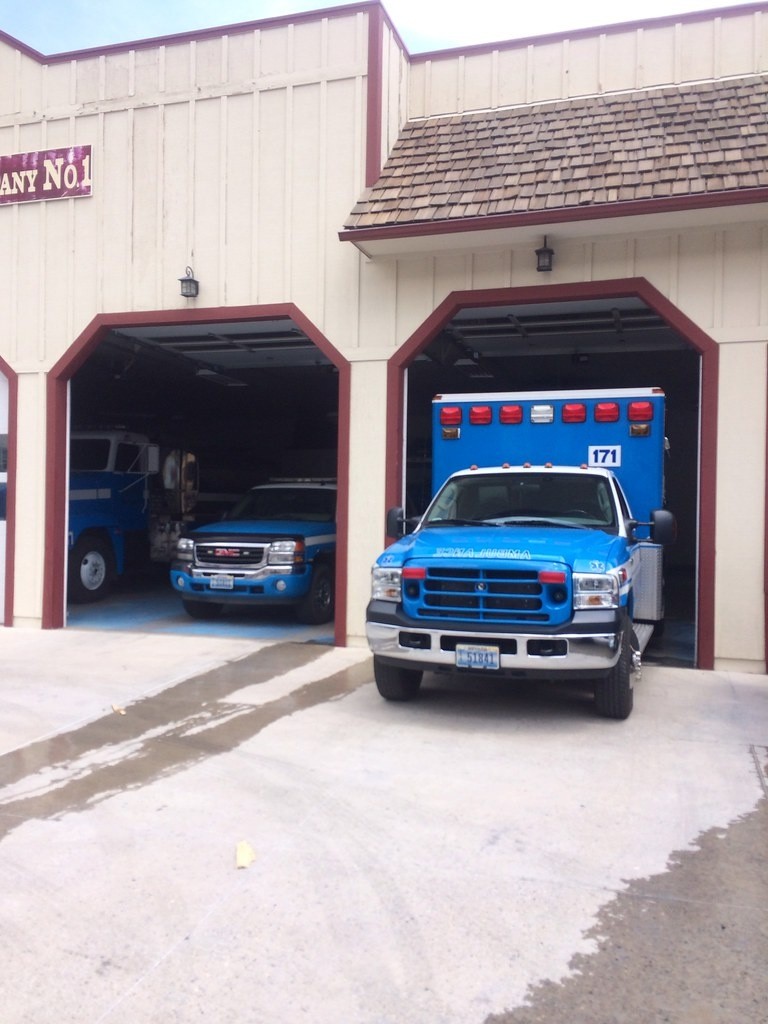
[535, 234, 554, 271]
[179, 266, 198, 298]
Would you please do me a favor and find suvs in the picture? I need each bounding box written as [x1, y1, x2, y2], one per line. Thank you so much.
[168, 475, 338, 626]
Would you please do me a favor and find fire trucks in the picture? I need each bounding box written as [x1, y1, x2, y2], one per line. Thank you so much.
[365, 387, 677, 722]
[1, 426, 161, 606]
